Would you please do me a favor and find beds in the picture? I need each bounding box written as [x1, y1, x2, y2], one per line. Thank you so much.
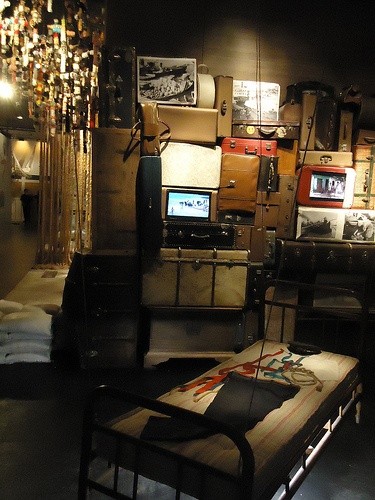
[76, 281, 370, 500]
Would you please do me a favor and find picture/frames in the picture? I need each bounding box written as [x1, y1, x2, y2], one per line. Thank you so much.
[136, 56, 197, 105]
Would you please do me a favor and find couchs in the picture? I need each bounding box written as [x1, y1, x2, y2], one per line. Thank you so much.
[0, 244, 70, 400]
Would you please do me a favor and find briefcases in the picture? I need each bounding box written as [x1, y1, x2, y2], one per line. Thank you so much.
[85, 64, 374, 273]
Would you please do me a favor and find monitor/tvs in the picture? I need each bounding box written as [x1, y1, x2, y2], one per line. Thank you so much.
[165, 189, 212, 222]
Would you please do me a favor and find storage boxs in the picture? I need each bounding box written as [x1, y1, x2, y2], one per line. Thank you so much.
[139, 75, 375, 308]
